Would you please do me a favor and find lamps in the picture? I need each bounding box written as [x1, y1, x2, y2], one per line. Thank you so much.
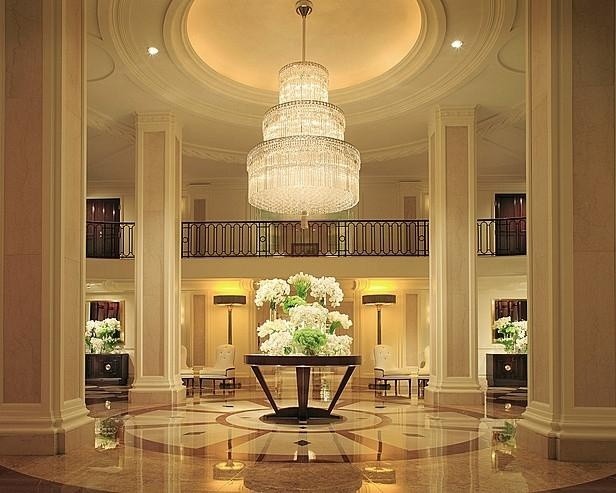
[360, 293, 396, 390]
[240, 1, 365, 229]
[210, 294, 247, 388]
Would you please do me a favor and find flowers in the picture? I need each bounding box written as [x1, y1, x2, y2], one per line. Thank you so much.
[250, 270, 356, 355]
[490, 314, 528, 354]
[83, 315, 125, 355]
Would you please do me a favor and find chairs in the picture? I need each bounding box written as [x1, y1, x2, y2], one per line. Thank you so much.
[180, 343, 195, 389]
[197, 344, 238, 394]
[417, 344, 432, 399]
[374, 343, 413, 399]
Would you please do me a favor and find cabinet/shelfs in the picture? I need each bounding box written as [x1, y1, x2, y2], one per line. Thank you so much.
[85, 352, 129, 386]
[485, 353, 527, 389]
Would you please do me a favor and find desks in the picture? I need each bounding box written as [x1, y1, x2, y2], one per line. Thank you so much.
[242, 422, 363, 491]
[240, 355, 364, 426]
